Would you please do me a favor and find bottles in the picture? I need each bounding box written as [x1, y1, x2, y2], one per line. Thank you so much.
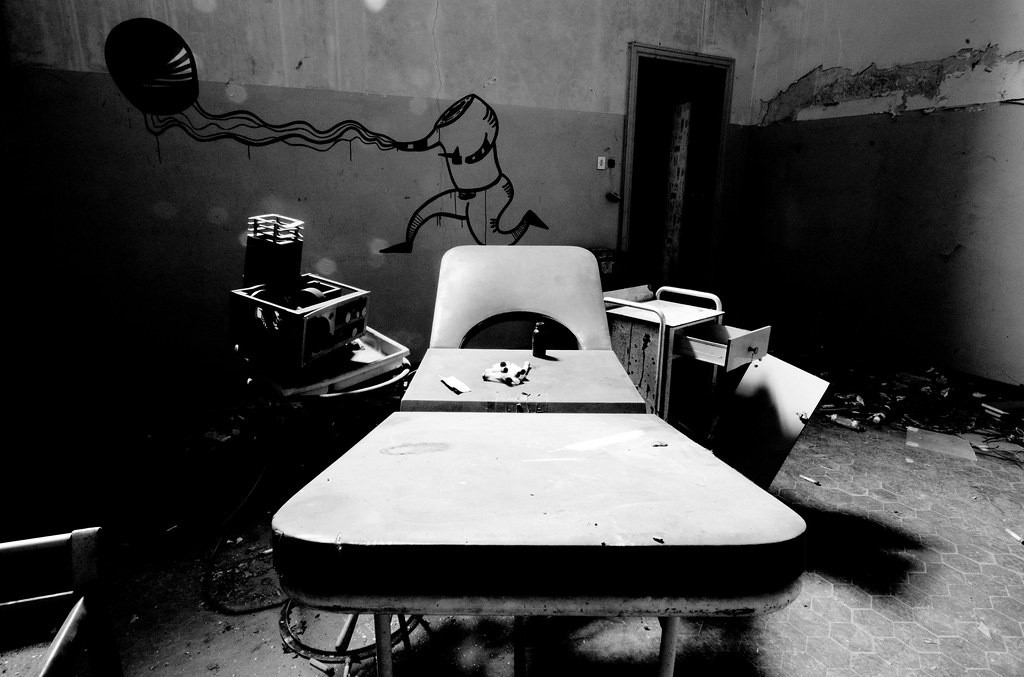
[532, 323, 546, 357]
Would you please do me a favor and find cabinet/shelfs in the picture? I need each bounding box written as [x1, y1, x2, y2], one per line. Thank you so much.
[603, 281, 833, 491]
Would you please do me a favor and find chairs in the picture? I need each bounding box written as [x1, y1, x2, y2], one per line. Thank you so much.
[270, 245, 808, 677]
[284, 368, 411, 651]
